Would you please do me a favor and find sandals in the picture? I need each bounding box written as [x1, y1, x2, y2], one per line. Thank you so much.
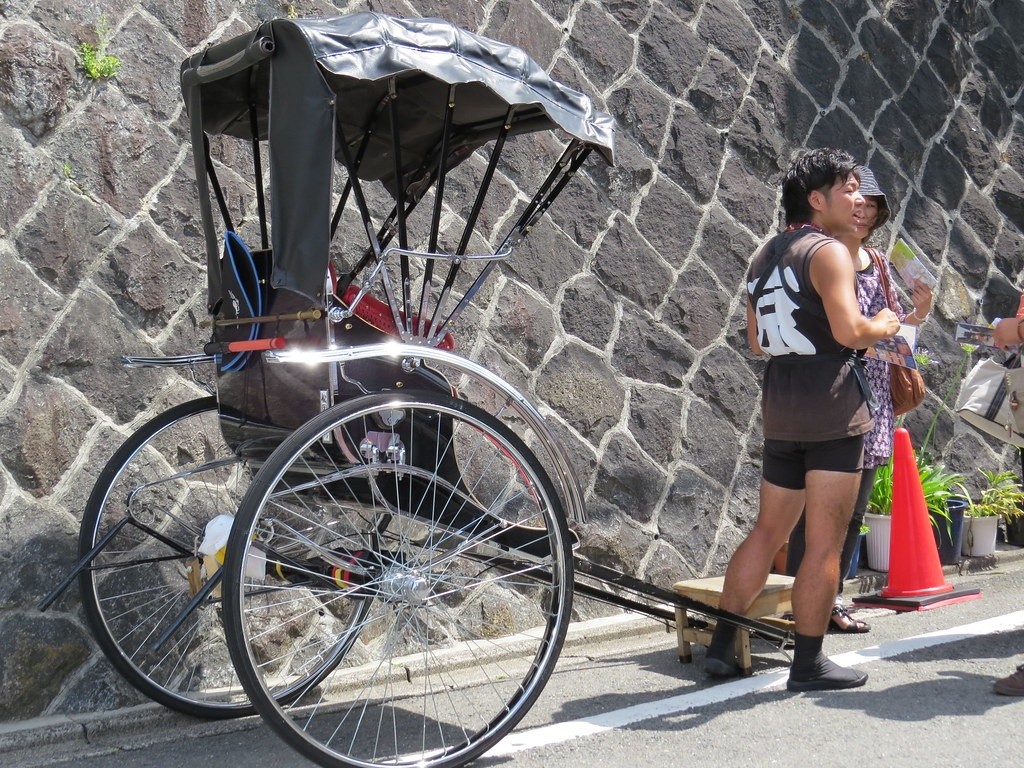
[828, 607, 870, 632]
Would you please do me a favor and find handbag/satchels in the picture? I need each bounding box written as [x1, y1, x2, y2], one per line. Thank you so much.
[891, 364, 924, 415]
[1003, 353, 1024, 435]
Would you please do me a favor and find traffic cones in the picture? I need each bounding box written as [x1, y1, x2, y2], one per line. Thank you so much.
[852, 428, 982, 612]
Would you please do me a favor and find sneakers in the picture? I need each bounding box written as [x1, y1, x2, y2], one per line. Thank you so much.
[993, 664, 1023, 696]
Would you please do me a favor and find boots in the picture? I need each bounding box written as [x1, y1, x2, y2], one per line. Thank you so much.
[787, 632, 869, 693]
[704, 621, 739, 677]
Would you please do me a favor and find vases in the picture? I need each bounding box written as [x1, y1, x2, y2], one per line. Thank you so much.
[928, 498, 970, 565]
[775, 542, 791, 575]
[847, 523, 869, 578]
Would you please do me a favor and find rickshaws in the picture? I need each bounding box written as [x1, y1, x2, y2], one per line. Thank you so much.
[73, 9, 812, 766]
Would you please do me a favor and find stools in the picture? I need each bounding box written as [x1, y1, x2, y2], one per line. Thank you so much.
[672, 571, 796, 676]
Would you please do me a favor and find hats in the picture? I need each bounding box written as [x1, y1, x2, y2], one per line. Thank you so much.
[855, 164, 891, 228]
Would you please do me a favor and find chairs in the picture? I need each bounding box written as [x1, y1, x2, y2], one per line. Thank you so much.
[213, 248, 475, 531]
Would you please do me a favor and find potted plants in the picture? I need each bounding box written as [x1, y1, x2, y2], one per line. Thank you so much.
[862, 447, 963, 573]
[961, 466, 1024, 558]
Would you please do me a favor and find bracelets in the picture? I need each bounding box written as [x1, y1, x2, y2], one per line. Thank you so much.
[1016, 320, 1023, 344]
[912, 308, 930, 320]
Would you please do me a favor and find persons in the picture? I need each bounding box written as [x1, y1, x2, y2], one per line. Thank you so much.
[785, 165, 932, 635]
[703, 145, 869, 695]
[993, 293, 1024, 699]
